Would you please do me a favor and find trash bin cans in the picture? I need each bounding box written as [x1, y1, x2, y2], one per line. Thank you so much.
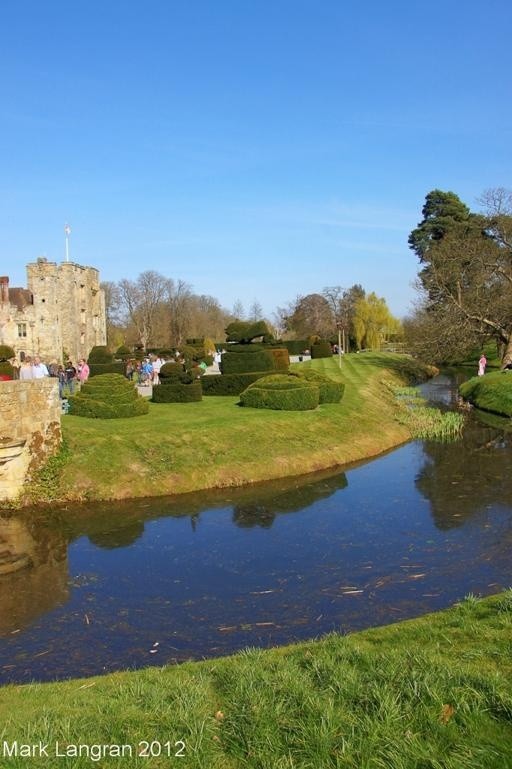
[299, 357, 303, 361]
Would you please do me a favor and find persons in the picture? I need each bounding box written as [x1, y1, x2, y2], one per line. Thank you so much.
[503, 354, 512, 370]
[19, 355, 90, 399]
[478, 355, 486, 376]
[208, 348, 227, 371]
[126, 357, 166, 387]
